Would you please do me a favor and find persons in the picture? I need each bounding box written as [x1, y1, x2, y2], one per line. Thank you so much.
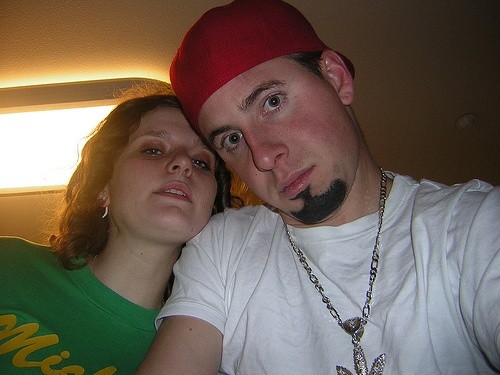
[136, 0, 500, 375]
[0, 91, 233, 375]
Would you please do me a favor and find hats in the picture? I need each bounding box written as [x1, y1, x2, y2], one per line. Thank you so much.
[169, 0, 356, 141]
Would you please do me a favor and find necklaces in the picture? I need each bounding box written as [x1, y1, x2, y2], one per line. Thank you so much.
[279, 167, 387, 375]
